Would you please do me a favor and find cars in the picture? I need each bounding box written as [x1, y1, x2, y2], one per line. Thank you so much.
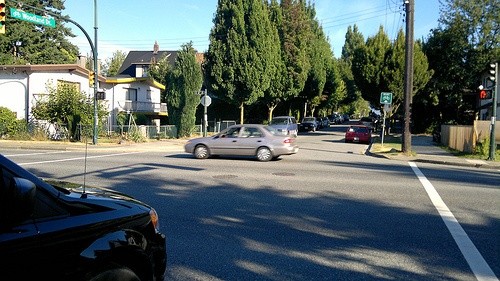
[344, 124, 372, 145]
[184, 124, 299, 162]
[300, 116, 317, 132]
[358, 112, 388, 133]
[266, 116, 299, 137]
[0, 152, 168, 281]
[316, 112, 350, 130]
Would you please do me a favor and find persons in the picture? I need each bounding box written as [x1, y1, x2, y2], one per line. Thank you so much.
[225, 129, 240, 138]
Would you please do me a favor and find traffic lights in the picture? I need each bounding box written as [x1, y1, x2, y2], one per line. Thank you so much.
[0, 0, 6, 34]
[479, 90, 492, 99]
[488, 62, 497, 83]
[89, 70, 95, 87]
[97, 92, 105, 100]
